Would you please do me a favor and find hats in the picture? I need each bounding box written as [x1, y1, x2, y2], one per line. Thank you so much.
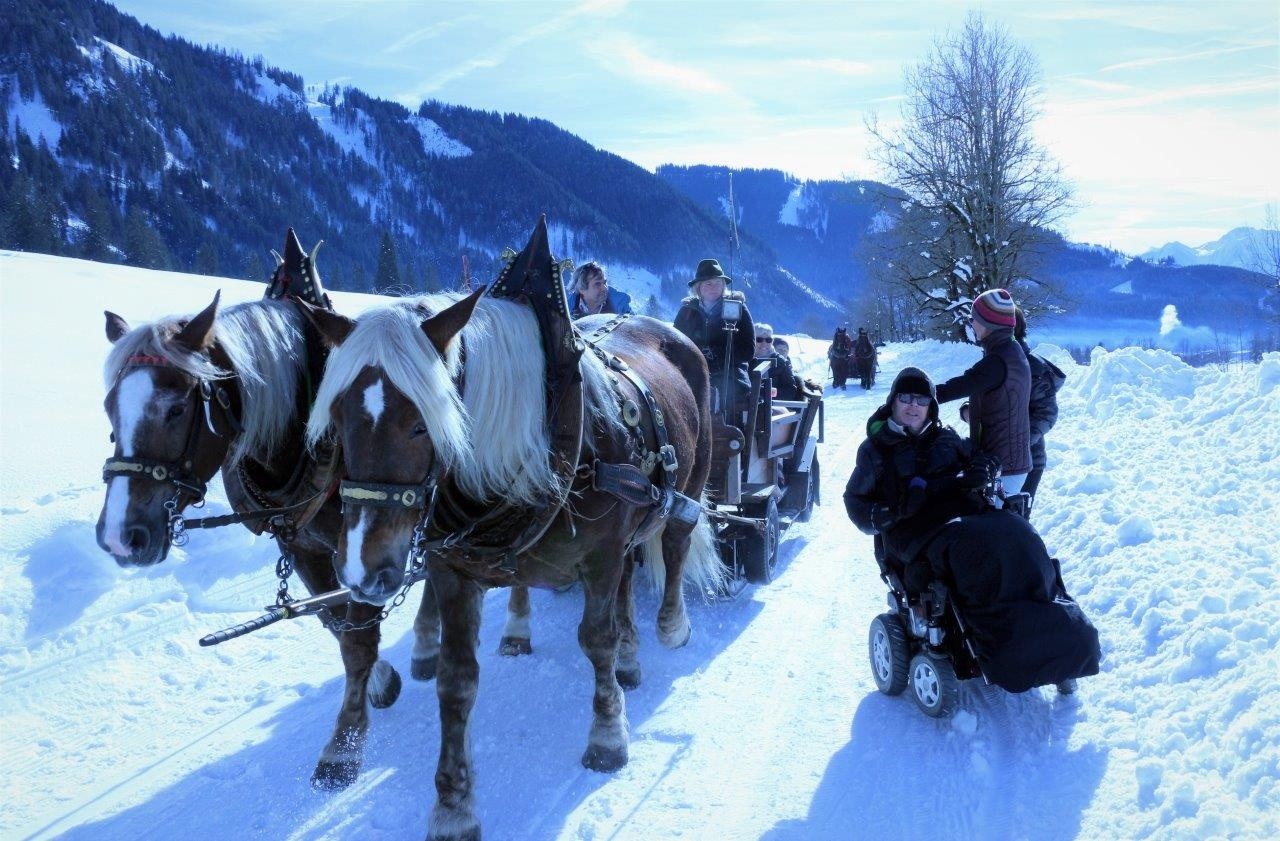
[1014, 307, 1027, 338]
[773, 337, 789, 347]
[688, 259, 733, 286]
[970, 289, 1017, 329]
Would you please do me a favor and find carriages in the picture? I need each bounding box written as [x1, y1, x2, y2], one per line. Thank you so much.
[90, 214, 825, 810]
[830, 338, 878, 390]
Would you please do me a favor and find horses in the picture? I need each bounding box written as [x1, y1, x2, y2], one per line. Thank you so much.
[293, 284, 738, 840]
[829, 326, 851, 391]
[93, 290, 534, 792]
[855, 327, 876, 390]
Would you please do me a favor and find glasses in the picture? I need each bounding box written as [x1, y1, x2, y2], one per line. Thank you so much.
[968, 317, 975, 324]
[756, 337, 773, 343]
[898, 393, 932, 406]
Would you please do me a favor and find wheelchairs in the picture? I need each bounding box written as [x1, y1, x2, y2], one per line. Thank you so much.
[866, 461, 1101, 720]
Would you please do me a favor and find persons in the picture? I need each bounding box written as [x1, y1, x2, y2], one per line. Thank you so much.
[673, 258, 823, 489]
[563, 260, 634, 326]
[843, 367, 1079, 694]
[934, 288, 1068, 523]
